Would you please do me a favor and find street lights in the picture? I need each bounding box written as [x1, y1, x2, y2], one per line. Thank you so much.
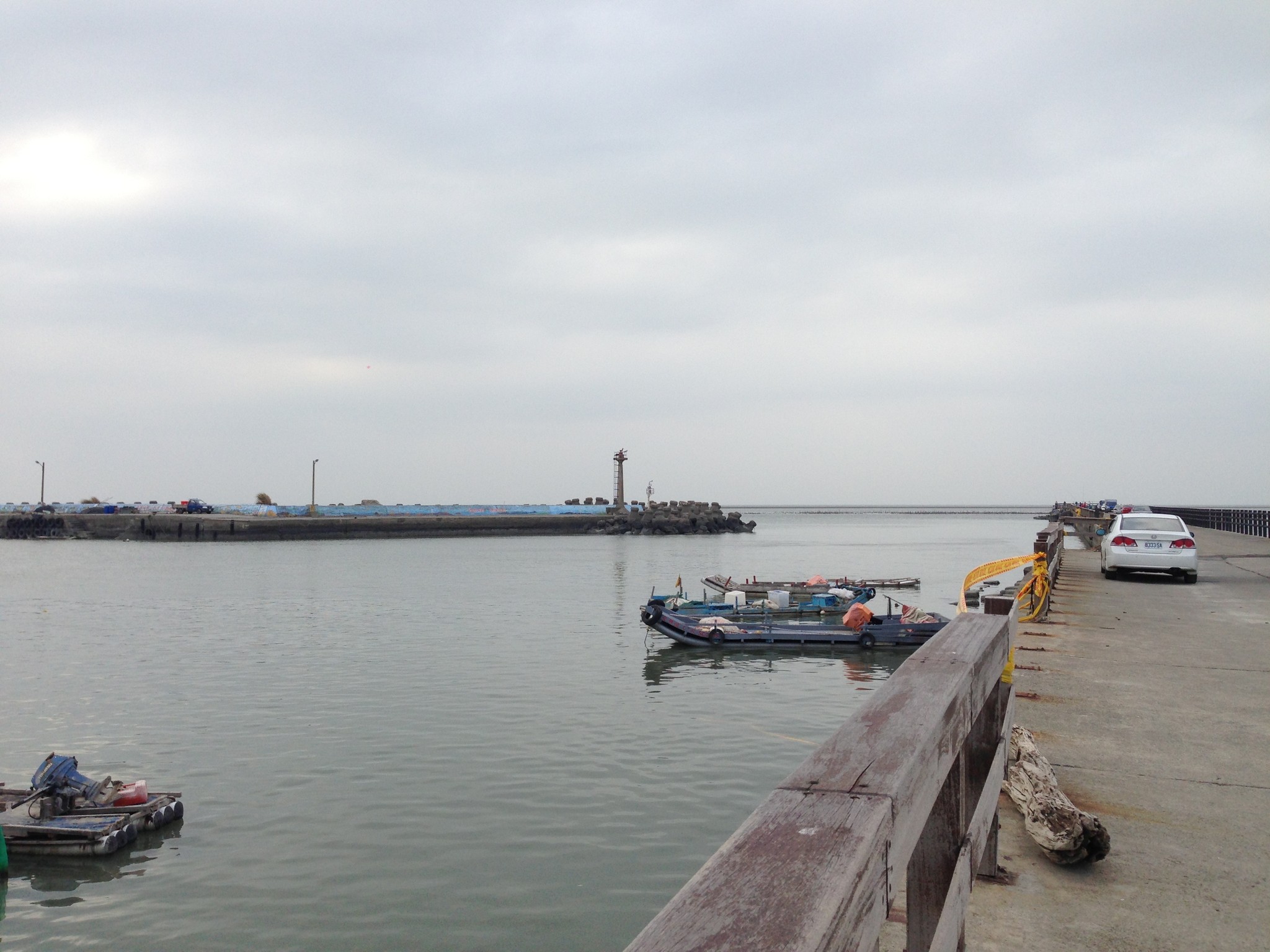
[312, 459, 319, 505]
[36, 461, 44, 502]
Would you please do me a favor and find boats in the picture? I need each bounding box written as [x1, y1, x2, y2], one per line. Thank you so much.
[0, 752, 183, 856]
[639, 573, 952, 649]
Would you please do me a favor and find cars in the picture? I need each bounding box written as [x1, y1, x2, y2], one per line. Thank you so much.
[1097, 503, 1100, 509]
[1130, 505, 1152, 513]
[1096, 513, 1197, 585]
[1101, 504, 1105, 512]
[1121, 505, 1134, 514]
[1113, 505, 1125, 514]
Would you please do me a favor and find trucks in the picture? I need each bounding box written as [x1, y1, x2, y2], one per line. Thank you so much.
[1105, 500, 1117, 513]
[172, 499, 214, 514]
[1099, 500, 1105, 509]
